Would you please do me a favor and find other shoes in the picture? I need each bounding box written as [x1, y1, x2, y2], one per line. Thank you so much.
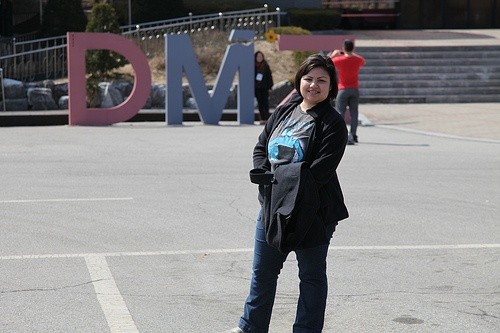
[222, 327, 247, 333]
[346, 135, 358, 145]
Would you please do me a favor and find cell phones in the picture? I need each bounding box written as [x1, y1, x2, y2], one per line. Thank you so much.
[338, 51, 345, 55]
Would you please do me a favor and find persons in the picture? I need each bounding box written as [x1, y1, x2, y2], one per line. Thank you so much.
[255, 51, 273, 120]
[328, 40, 367, 145]
[219, 55, 348, 333]
